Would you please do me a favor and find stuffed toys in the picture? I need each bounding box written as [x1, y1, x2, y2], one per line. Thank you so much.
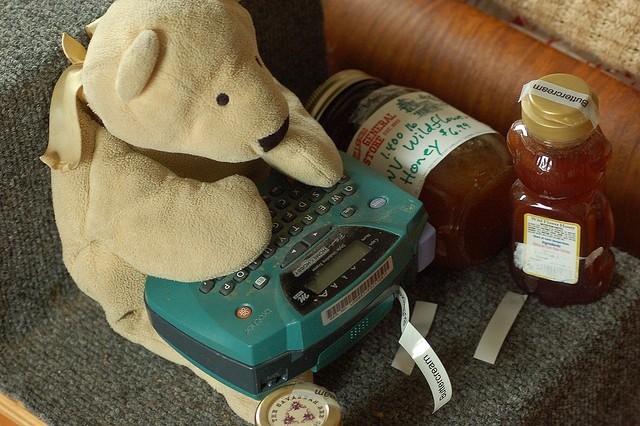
[39, 0, 344, 425]
